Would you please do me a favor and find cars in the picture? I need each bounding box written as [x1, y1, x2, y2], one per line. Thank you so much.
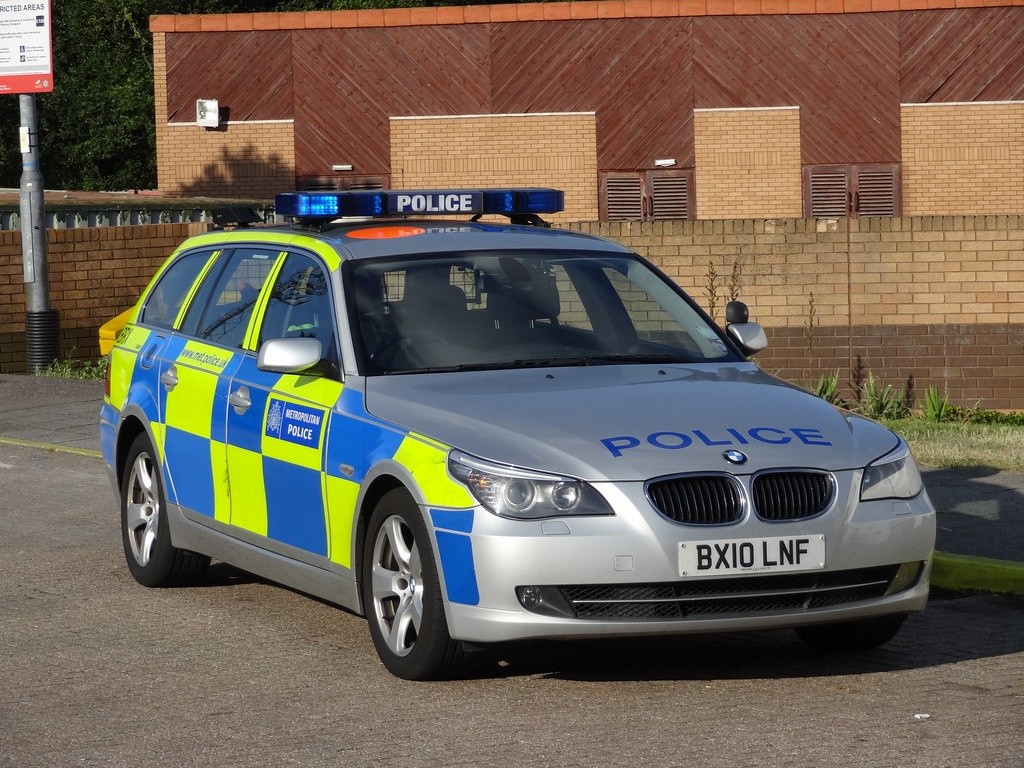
[100, 186, 939, 681]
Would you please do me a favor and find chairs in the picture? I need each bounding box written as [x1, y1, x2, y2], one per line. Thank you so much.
[413, 286, 468, 338]
[484, 274, 569, 361]
